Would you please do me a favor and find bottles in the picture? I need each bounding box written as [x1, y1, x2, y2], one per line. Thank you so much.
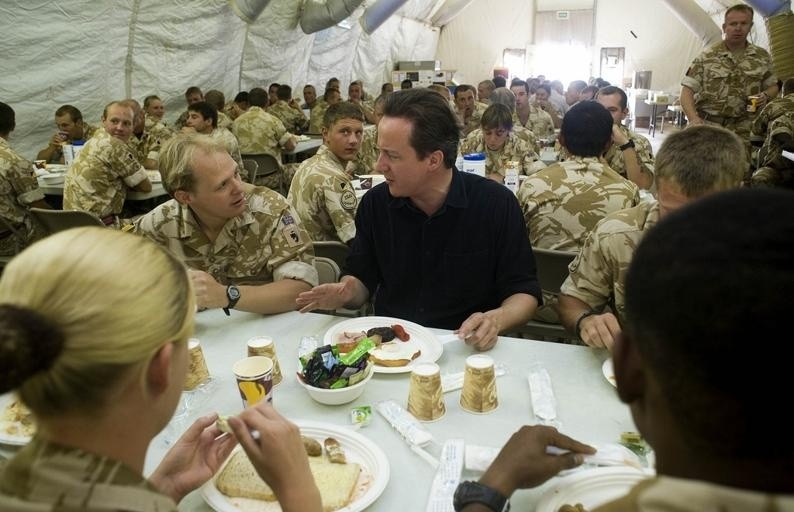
[63, 144, 73, 166]
[505, 161, 520, 194]
[71, 140, 85, 158]
[464, 152, 485, 177]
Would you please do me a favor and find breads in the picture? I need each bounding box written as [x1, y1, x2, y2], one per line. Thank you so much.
[301, 433, 321, 455]
[309, 454, 360, 511]
[326, 438, 345, 463]
[368, 344, 420, 367]
[3, 399, 35, 437]
[216, 446, 279, 501]
[336, 332, 364, 352]
[216, 415, 233, 434]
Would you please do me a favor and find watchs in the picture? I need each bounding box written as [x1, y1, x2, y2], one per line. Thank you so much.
[620, 138, 635, 152]
[221, 284, 242, 317]
[451, 479, 513, 512]
[575, 309, 600, 342]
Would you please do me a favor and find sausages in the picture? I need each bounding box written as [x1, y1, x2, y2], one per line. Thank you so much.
[393, 324, 409, 341]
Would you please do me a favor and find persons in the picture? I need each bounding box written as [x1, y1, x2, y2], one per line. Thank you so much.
[121, 132, 320, 314]
[477, 79, 496, 104]
[0, 101, 55, 257]
[224, 90, 249, 120]
[510, 80, 555, 145]
[308, 87, 341, 135]
[171, 85, 203, 130]
[121, 98, 165, 171]
[375, 82, 393, 102]
[467, 87, 540, 156]
[268, 82, 281, 106]
[554, 123, 744, 349]
[461, 103, 548, 187]
[204, 89, 234, 130]
[347, 78, 376, 125]
[530, 83, 564, 129]
[232, 87, 302, 189]
[458, 186, 794, 512]
[452, 84, 490, 138]
[61, 100, 153, 231]
[1, 226, 324, 512]
[180, 101, 249, 184]
[266, 84, 309, 135]
[285, 101, 364, 245]
[679, 3, 779, 133]
[515, 98, 641, 324]
[293, 86, 545, 352]
[359, 92, 390, 175]
[318, 77, 343, 103]
[300, 85, 319, 110]
[750, 78, 794, 187]
[592, 86, 656, 190]
[36, 104, 98, 166]
[141, 94, 173, 151]
[564, 79, 588, 114]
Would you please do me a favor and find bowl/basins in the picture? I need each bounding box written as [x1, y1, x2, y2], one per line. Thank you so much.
[42, 173, 66, 185]
[293, 346, 374, 406]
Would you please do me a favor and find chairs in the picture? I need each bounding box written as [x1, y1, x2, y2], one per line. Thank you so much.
[531, 246, 580, 299]
[316, 257, 341, 286]
[27, 206, 106, 235]
[311, 240, 375, 317]
[242, 157, 258, 185]
[241, 153, 289, 196]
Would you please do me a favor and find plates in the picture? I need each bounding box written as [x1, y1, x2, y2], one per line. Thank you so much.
[147, 170, 161, 184]
[601, 357, 620, 391]
[1, 391, 33, 446]
[200, 421, 390, 512]
[323, 316, 444, 374]
[534, 467, 656, 510]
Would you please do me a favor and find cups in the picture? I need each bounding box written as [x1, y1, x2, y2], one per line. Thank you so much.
[246, 336, 284, 388]
[186, 338, 213, 390]
[407, 361, 446, 421]
[358, 174, 373, 189]
[235, 357, 274, 411]
[458, 354, 498, 413]
